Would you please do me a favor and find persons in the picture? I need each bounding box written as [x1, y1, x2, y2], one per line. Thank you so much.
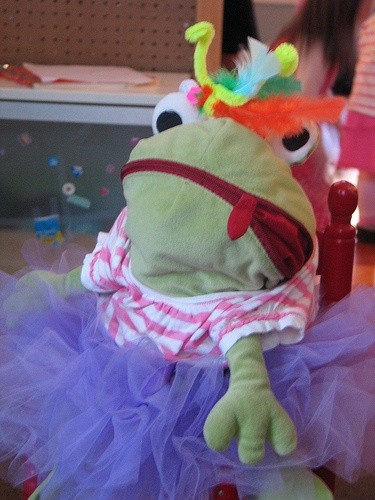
[269, 0, 364, 230]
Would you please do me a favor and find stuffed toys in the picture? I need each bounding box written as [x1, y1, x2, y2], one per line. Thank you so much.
[1, 21, 375, 499]
